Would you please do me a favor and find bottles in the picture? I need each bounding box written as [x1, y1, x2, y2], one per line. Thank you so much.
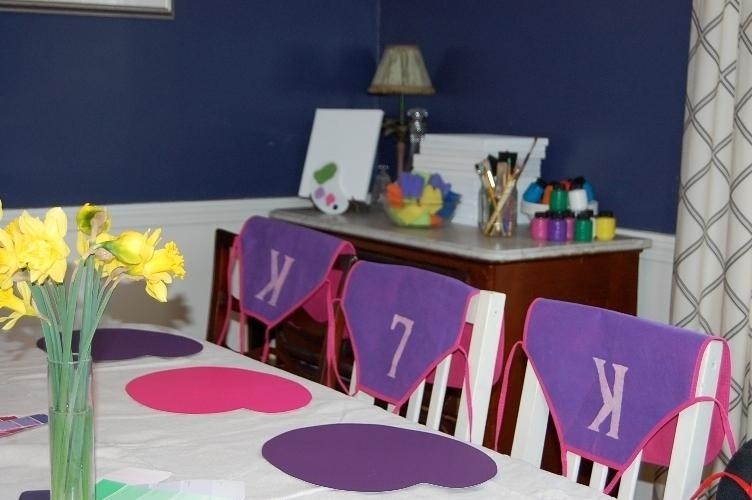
[523, 175, 617, 242]
[372, 167, 392, 210]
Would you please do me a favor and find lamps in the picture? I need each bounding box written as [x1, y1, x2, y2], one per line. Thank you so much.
[364, 41, 441, 179]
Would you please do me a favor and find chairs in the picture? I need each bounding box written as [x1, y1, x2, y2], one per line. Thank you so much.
[509, 296, 726, 500]
[344, 264, 509, 451]
[205, 226, 355, 395]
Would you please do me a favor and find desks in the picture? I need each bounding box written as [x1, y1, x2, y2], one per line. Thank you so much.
[0, 312, 623, 500]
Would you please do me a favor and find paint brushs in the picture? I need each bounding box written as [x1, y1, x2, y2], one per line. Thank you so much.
[475, 132, 539, 236]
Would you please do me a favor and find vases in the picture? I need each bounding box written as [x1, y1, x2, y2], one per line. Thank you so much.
[47, 350, 98, 500]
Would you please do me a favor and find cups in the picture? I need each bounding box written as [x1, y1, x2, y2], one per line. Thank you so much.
[478, 186, 518, 238]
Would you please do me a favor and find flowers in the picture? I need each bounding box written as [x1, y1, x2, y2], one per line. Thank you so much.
[0, 195, 186, 500]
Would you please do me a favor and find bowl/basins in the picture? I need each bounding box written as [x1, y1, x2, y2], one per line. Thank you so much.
[376, 201, 462, 228]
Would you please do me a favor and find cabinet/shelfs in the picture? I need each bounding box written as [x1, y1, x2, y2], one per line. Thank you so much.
[268, 208, 655, 475]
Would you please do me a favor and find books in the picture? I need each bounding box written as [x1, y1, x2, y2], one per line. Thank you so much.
[409, 129, 549, 228]
